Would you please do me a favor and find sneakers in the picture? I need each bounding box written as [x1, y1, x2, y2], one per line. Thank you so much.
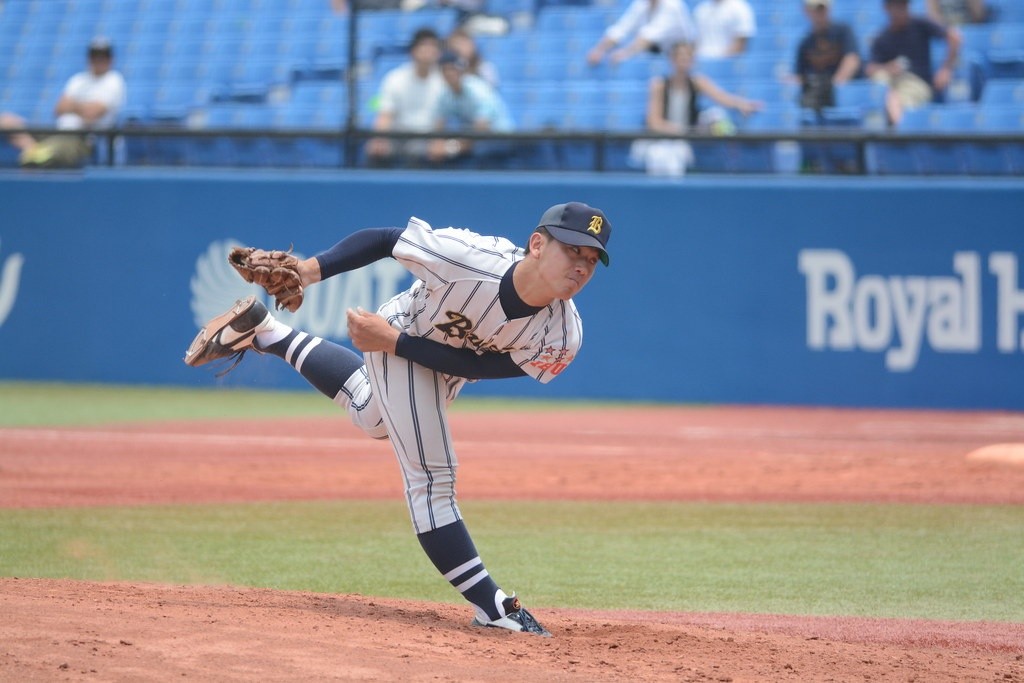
[185, 294, 275, 378]
[472, 589, 552, 637]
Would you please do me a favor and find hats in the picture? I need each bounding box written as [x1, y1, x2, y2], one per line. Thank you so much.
[535, 202, 611, 267]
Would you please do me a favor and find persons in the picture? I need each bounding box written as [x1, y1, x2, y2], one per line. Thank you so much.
[926, 0, 990, 25]
[55, 35, 126, 165]
[0, 110, 61, 167]
[182, 200, 613, 636]
[774, 0, 862, 119]
[365, 25, 518, 168]
[865, 0, 963, 137]
[585, 0, 757, 71]
[627, 41, 767, 179]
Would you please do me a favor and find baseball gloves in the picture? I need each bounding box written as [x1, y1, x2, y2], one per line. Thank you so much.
[226, 246, 305, 313]
